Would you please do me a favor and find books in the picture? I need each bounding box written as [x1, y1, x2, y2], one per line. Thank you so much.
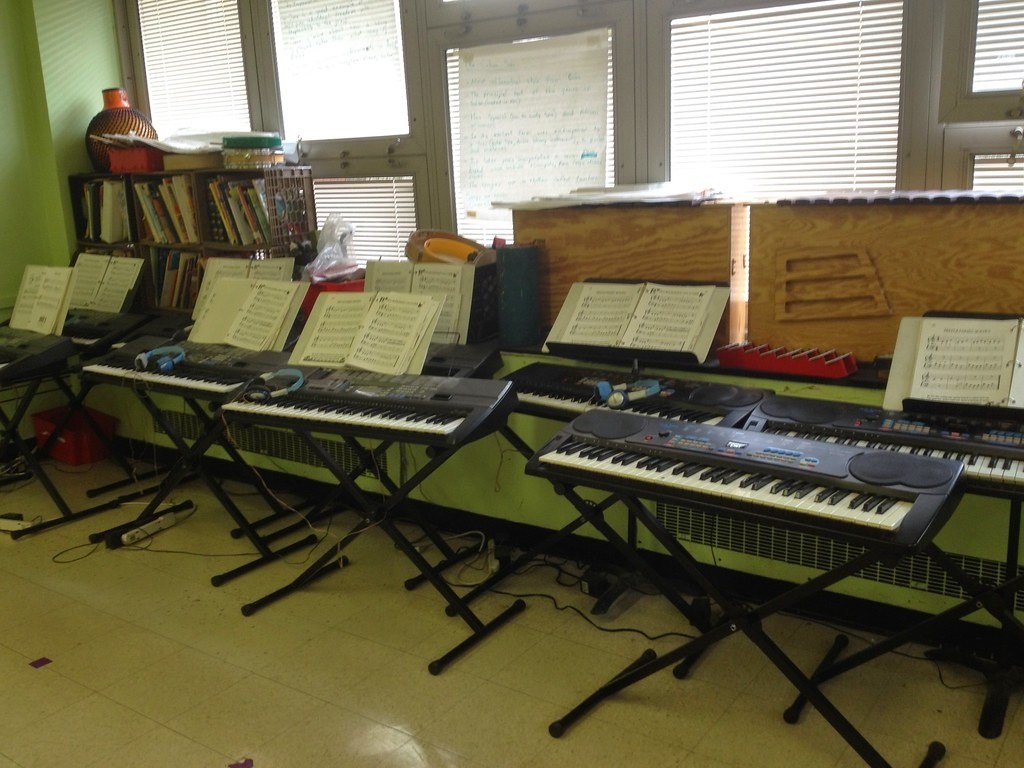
[79, 180, 131, 244]
[189, 255, 295, 319]
[187, 276, 310, 350]
[85, 247, 246, 310]
[880, 316, 1023, 411]
[70, 252, 148, 315]
[205, 173, 294, 247]
[8, 263, 79, 337]
[541, 279, 731, 364]
[364, 259, 475, 346]
[133, 174, 200, 244]
[288, 292, 446, 375]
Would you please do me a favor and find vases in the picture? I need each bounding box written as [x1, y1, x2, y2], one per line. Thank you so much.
[85, 87, 159, 173]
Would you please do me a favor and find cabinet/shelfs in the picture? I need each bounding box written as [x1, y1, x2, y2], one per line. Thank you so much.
[68, 166, 316, 310]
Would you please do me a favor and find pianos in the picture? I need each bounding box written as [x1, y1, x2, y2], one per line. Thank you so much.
[498, 362, 776, 432]
[522, 408, 967, 555]
[77, 311, 304, 399]
[222, 342, 519, 451]
[0, 308, 158, 387]
[740, 394, 1024, 495]
